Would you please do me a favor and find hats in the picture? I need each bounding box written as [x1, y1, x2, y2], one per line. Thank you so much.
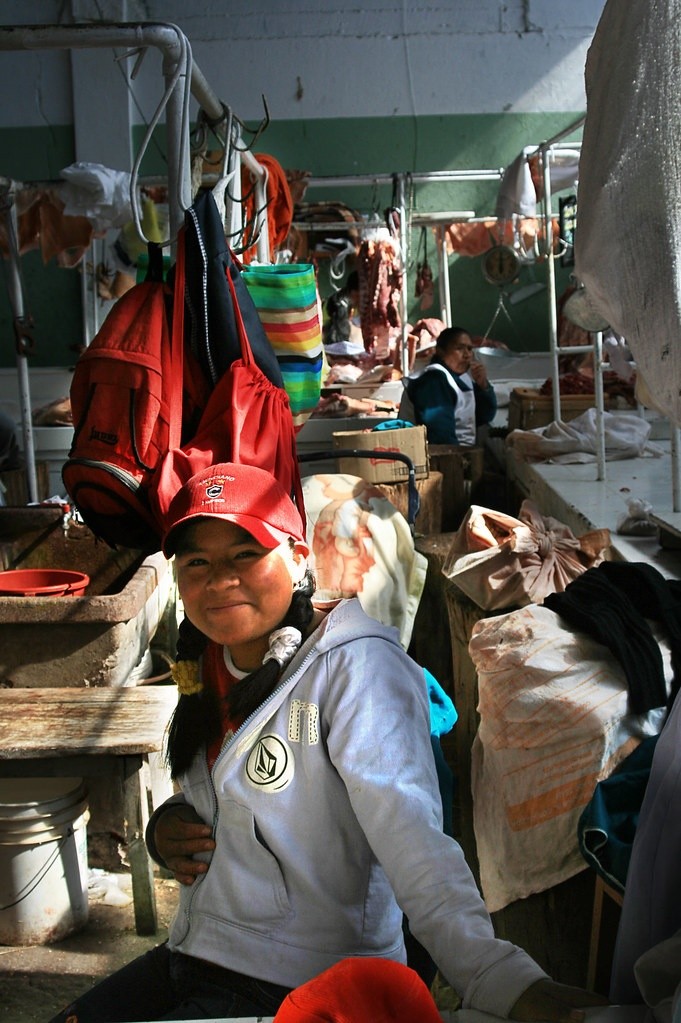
[162, 463, 304, 560]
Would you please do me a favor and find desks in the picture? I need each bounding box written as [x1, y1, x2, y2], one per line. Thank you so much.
[1, 686, 182, 934]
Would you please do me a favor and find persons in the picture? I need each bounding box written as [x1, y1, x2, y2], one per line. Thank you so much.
[400, 327, 512, 511]
[0, 413, 21, 471]
[47, 463, 609, 1023]
[321, 271, 359, 343]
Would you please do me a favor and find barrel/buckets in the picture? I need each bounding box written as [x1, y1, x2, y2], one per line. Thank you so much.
[0, 775, 91, 948]
[0, 568, 90, 597]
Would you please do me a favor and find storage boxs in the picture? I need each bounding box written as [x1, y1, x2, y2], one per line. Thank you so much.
[331, 425, 430, 485]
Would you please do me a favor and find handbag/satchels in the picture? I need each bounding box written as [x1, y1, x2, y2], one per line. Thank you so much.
[228, 248, 322, 431]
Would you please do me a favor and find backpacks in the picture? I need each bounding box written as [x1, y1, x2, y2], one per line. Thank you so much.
[151, 228, 307, 538]
[61, 240, 204, 554]
